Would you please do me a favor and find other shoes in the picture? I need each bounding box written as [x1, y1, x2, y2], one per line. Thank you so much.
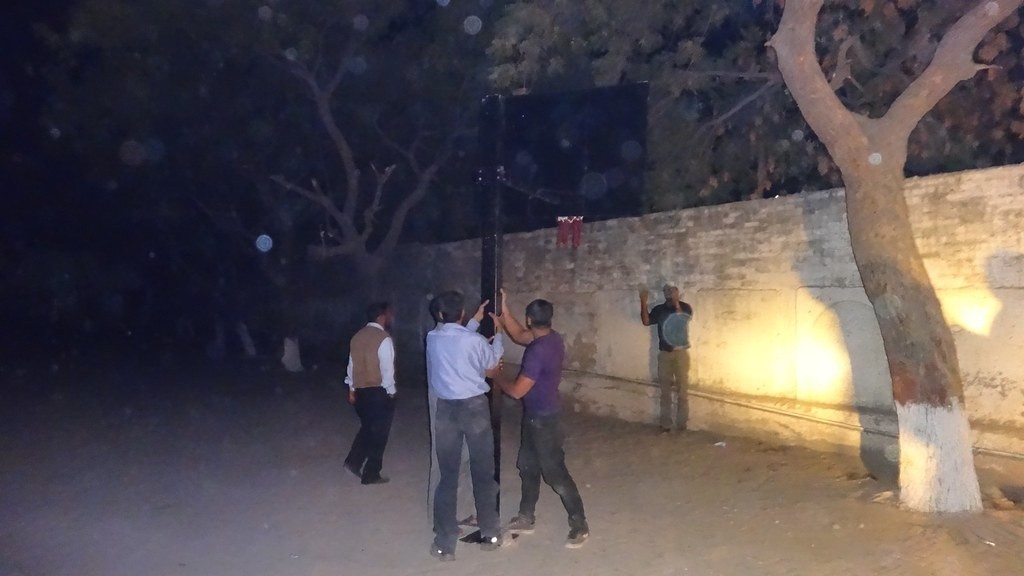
[457, 514, 480, 529]
[659, 426, 670, 436]
[679, 424, 687, 436]
[361, 473, 391, 486]
[343, 463, 362, 479]
[430, 543, 455, 561]
[481, 533, 503, 551]
[457, 527, 464, 538]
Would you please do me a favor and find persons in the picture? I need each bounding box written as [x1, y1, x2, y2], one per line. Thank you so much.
[424, 291, 505, 563]
[344, 302, 397, 484]
[423, 288, 510, 538]
[637, 281, 693, 437]
[484, 289, 590, 545]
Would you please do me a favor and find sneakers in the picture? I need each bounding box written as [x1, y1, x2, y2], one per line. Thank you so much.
[503, 511, 536, 534]
[566, 521, 589, 549]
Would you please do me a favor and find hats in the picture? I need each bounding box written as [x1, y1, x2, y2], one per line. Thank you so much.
[662, 281, 678, 288]
